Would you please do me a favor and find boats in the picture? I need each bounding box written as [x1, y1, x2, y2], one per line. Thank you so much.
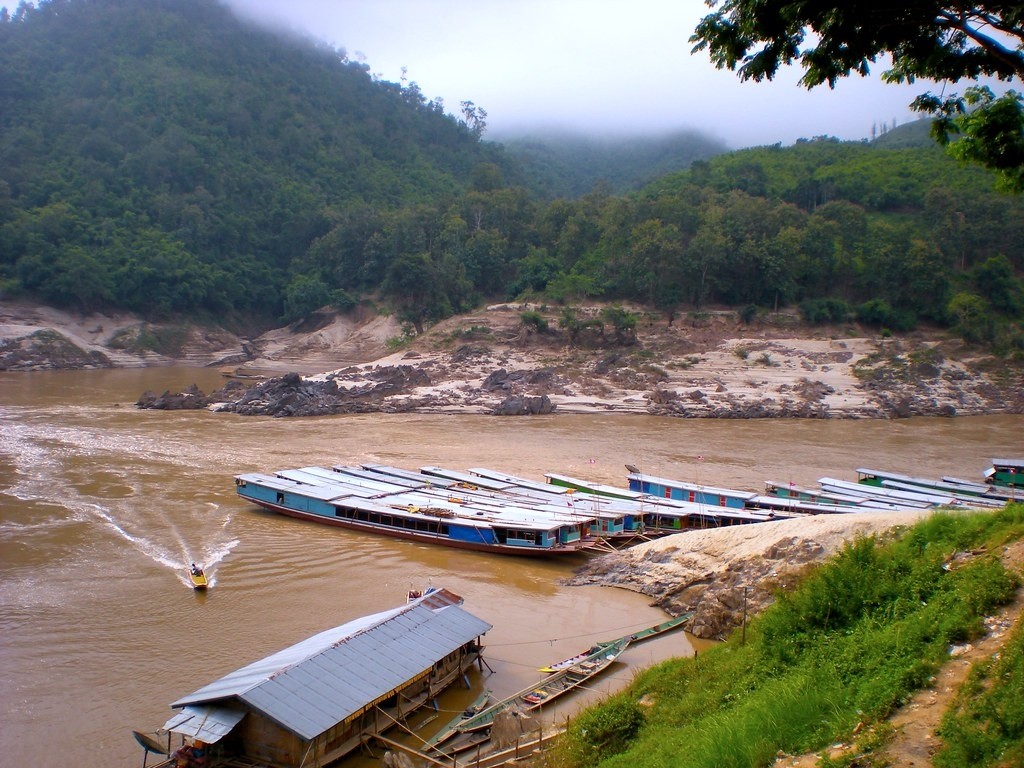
[454, 635, 634, 735]
[420, 688, 492, 751]
[235, 455, 1024, 559]
[220, 372, 270, 379]
[425, 724, 493, 760]
[189, 567, 208, 591]
[537, 610, 696, 674]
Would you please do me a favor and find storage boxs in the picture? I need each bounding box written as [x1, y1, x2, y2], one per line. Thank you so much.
[170, 739, 206, 768]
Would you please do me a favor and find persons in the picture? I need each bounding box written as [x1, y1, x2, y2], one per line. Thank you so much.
[192, 564, 202, 575]
